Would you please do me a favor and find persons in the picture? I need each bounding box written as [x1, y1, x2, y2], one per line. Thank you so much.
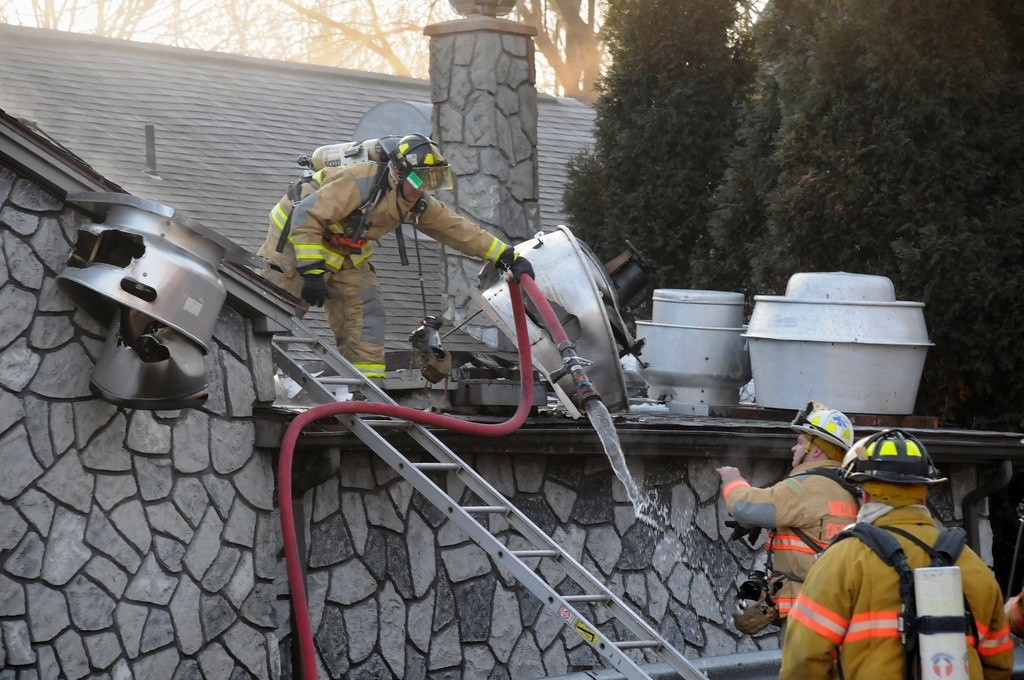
[256, 134, 534, 418]
[716, 400, 859, 679]
[781, 428, 1013, 680]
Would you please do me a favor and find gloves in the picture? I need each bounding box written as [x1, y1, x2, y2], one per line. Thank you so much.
[295, 262, 336, 308]
[724, 514, 761, 546]
[498, 249, 535, 283]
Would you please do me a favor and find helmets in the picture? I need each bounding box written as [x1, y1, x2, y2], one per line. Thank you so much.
[838, 429, 951, 487]
[389, 132, 449, 185]
[792, 398, 856, 452]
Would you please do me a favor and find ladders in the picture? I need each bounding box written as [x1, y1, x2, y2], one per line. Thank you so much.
[271, 313, 710, 680]
